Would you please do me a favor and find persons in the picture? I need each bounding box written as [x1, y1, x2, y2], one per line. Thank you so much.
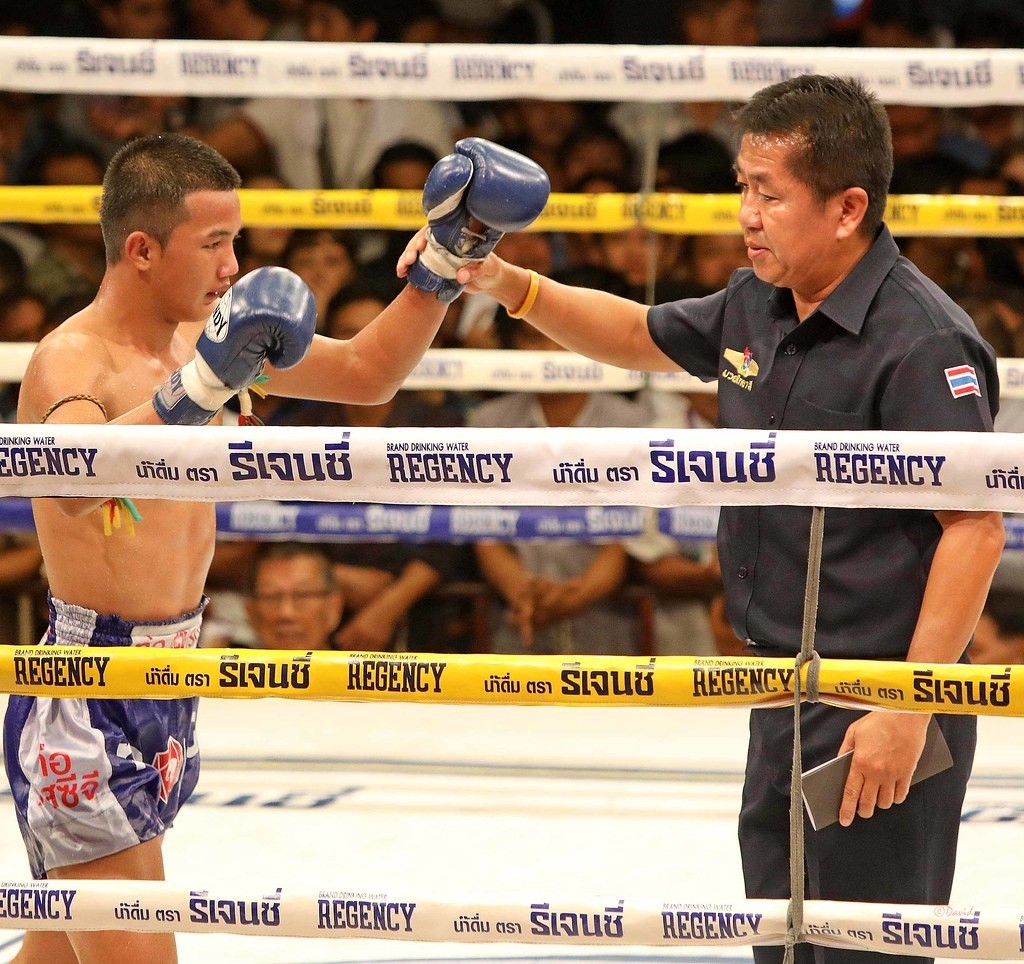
[397, 75, 1007, 964]
[5, 135, 552, 964]
[0, 0, 1024, 666]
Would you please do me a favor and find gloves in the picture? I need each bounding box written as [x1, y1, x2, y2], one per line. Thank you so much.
[408, 136, 552, 304]
[152, 266, 320, 429]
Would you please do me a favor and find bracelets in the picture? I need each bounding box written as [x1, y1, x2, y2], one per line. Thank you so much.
[506, 268, 540, 320]
